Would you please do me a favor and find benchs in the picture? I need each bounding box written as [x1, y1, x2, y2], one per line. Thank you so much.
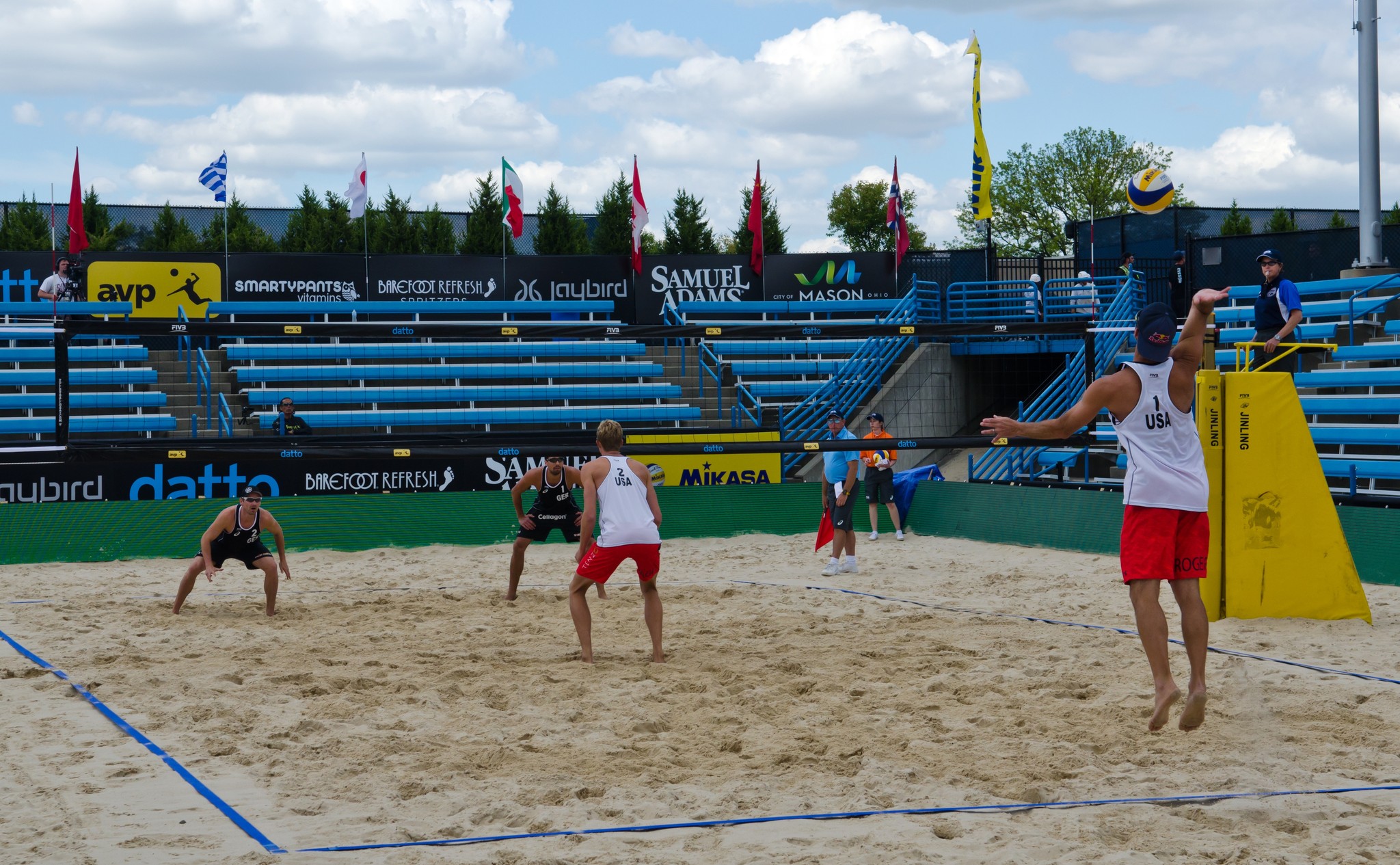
[0, 299, 919, 436]
[1032, 273, 1400, 502]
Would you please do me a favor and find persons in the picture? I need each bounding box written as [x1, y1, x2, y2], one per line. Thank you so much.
[978, 287, 1234, 733]
[171, 485, 292, 617]
[37, 257, 82, 321]
[860, 413, 904, 541]
[504, 456, 612, 600]
[821, 410, 862, 576]
[1026, 251, 1303, 387]
[273, 398, 312, 437]
[569, 420, 664, 664]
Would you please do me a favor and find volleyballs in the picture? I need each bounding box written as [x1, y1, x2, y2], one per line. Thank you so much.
[873, 450, 889, 463]
[1126, 168, 1174, 214]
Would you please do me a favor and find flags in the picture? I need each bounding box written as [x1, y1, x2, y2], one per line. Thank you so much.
[199, 151, 227, 202]
[886, 156, 909, 268]
[67, 149, 88, 252]
[747, 161, 762, 275]
[815, 506, 834, 553]
[501, 157, 524, 238]
[343, 154, 368, 219]
[964, 33, 993, 221]
[632, 156, 649, 274]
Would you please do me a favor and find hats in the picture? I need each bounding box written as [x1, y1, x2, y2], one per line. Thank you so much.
[826, 410, 844, 419]
[1137, 302, 1178, 363]
[1256, 249, 1283, 263]
[1121, 252, 1135, 261]
[240, 485, 262, 497]
[866, 413, 884, 423]
[1172, 250, 1185, 262]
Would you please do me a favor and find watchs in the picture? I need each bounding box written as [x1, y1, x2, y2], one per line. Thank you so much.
[1274, 334, 1281, 341]
[842, 489, 849, 495]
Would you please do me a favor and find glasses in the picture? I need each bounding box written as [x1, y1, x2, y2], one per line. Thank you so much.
[1129, 257, 1134, 259]
[281, 403, 293, 406]
[1260, 261, 1282, 267]
[1135, 309, 1142, 331]
[827, 418, 842, 424]
[241, 497, 262, 503]
[546, 457, 564, 463]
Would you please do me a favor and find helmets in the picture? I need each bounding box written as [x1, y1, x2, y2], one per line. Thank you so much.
[1030, 274, 1041, 282]
[1087, 273, 1091, 278]
[1078, 271, 1088, 278]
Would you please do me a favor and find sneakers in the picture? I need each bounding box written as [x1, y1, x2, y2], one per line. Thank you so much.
[821, 563, 840, 576]
[895, 532, 904, 541]
[839, 561, 859, 573]
[868, 531, 878, 541]
